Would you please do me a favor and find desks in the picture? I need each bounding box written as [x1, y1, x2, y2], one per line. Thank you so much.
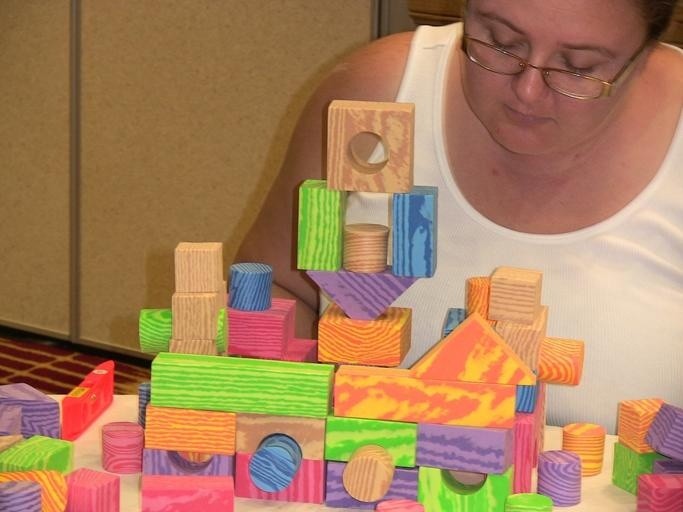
[47, 394, 637, 511]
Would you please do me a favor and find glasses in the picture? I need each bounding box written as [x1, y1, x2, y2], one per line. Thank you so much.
[459, 12, 659, 101]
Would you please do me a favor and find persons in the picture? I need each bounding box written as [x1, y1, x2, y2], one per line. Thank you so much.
[229, 0, 682, 430]
